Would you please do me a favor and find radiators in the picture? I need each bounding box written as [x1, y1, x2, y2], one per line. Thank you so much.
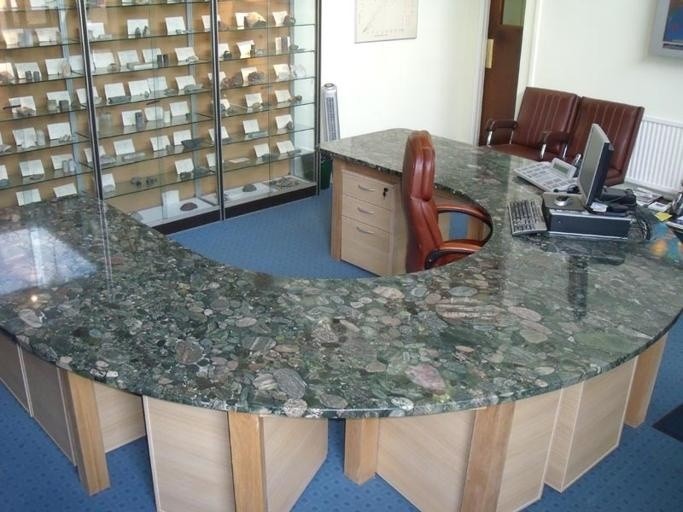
[621, 111, 680, 195]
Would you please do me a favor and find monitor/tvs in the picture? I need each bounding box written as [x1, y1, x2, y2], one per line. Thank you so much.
[576, 123, 615, 206]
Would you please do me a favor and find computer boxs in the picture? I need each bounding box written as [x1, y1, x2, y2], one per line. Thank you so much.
[543, 192, 631, 241]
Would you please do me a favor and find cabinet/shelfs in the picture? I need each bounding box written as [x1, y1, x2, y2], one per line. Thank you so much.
[0, 0, 320, 232]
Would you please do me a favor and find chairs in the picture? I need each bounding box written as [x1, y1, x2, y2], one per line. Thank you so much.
[402, 129, 490, 274]
[486, 87, 644, 189]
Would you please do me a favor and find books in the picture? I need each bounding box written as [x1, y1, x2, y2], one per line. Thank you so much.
[647, 195, 673, 212]
[629, 186, 662, 207]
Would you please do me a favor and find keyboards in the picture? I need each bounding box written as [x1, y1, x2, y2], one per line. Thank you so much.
[508, 198, 547, 237]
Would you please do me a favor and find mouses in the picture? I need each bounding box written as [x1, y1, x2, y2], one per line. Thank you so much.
[554, 195, 573, 207]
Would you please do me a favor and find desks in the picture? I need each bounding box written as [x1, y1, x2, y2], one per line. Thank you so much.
[1, 126, 681, 512]
